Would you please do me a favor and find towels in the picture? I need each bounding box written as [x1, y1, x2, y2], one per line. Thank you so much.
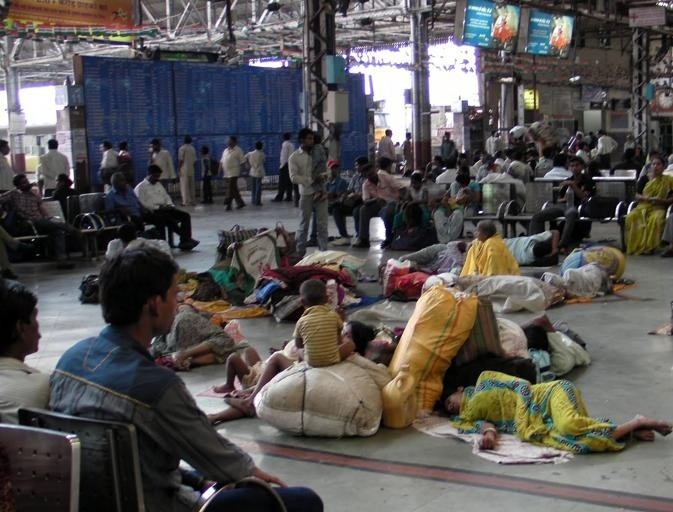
[413, 414, 572, 468]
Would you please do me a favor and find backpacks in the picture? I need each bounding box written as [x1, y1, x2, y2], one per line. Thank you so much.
[203, 157, 219, 175]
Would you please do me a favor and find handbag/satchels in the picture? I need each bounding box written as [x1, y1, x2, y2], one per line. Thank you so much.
[583, 196, 618, 223]
[454, 298, 506, 366]
[216, 223, 268, 260]
[228, 227, 281, 292]
[273, 222, 295, 253]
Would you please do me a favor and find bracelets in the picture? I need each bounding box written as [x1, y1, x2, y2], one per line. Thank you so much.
[482, 427, 497, 435]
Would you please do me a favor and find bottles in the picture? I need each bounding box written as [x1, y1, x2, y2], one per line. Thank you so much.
[565, 185, 574, 209]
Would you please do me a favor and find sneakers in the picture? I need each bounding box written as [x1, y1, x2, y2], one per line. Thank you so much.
[56, 262, 72, 268]
[2, 269, 17, 279]
[224, 204, 231, 210]
[305, 237, 317, 245]
[331, 238, 350, 246]
[353, 237, 369, 249]
[284, 195, 292, 201]
[235, 204, 246, 209]
[16, 242, 33, 252]
[200, 199, 213, 204]
[271, 194, 283, 201]
[661, 245, 673, 257]
[179, 239, 199, 249]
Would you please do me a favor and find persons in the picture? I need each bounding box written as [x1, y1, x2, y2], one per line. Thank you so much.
[51, 173, 78, 218]
[329, 136, 383, 248]
[0, 280, 53, 427]
[310, 132, 329, 204]
[106, 171, 151, 237]
[97, 222, 139, 302]
[155, 301, 234, 371]
[244, 140, 266, 207]
[1, 173, 82, 270]
[288, 128, 328, 259]
[100, 168, 115, 194]
[47, 244, 323, 512]
[97, 141, 120, 168]
[117, 140, 131, 162]
[444, 366, 673, 455]
[217, 136, 247, 211]
[384, 117, 673, 354]
[148, 139, 176, 191]
[200, 145, 214, 204]
[213, 322, 370, 394]
[37, 138, 70, 198]
[271, 133, 296, 202]
[177, 136, 196, 206]
[0, 221, 35, 279]
[0, 139, 20, 194]
[204, 351, 295, 425]
[119, 163, 144, 189]
[294, 278, 355, 368]
[133, 164, 200, 251]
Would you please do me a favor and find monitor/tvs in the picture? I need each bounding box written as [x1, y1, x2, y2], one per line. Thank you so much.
[526, 7, 575, 59]
[461, 0, 522, 55]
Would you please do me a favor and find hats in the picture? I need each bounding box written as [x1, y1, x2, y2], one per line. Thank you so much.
[326, 160, 338, 168]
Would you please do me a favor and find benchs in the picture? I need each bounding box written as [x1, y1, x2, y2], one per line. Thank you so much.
[78, 192, 173, 257]
[2, 200, 99, 258]
[503, 180, 629, 252]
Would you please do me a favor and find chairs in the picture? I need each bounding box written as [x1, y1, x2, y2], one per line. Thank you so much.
[18, 405, 287, 512]
[462, 179, 511, 254]
[1, 424, 81, 512]
